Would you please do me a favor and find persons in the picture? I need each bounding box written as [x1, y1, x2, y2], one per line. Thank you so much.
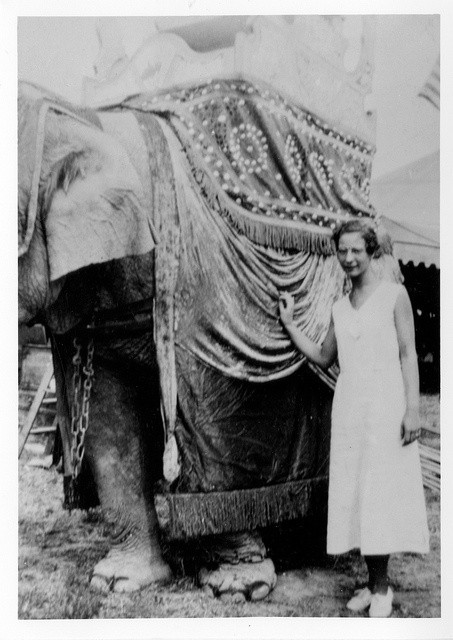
[277, 219, 430, 617]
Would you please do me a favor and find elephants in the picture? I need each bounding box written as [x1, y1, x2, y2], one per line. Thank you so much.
[17, 78, 400, 605]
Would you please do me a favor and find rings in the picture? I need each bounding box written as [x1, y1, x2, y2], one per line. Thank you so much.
[415, 433, 419, 437]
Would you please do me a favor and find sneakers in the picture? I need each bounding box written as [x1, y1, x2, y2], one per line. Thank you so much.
[345, 585, 372, 611]
[368, 585, 393, 617]
